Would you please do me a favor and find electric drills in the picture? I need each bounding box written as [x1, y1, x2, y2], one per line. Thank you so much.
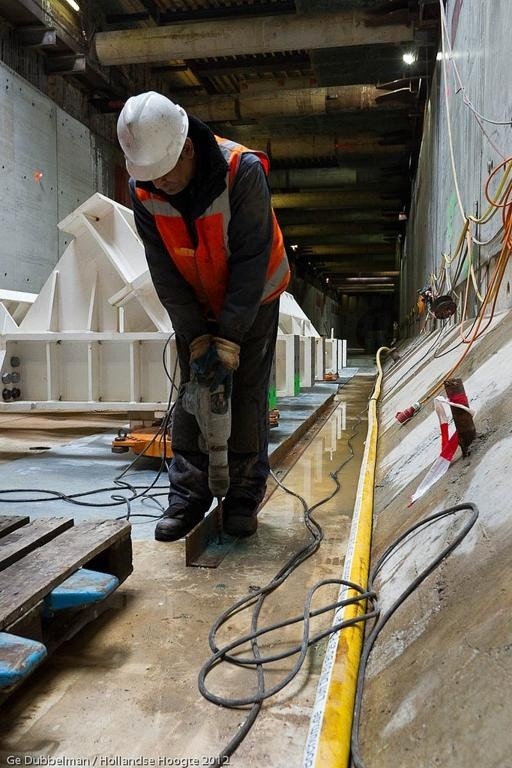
[181, 357, 234, 546]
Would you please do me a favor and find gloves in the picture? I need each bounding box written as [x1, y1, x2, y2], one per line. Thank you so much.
[188, 334, 211, 385]
[206, 335, 242, 399]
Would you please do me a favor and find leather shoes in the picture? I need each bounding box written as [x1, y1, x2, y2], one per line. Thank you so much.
[154, 513, 206, 542]
[222, 513, 258, 537]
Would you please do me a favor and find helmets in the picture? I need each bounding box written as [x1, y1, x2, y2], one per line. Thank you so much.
[117, 90, 191, 182]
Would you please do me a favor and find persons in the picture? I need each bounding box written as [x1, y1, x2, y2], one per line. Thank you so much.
[116, 90, 291, 542]
